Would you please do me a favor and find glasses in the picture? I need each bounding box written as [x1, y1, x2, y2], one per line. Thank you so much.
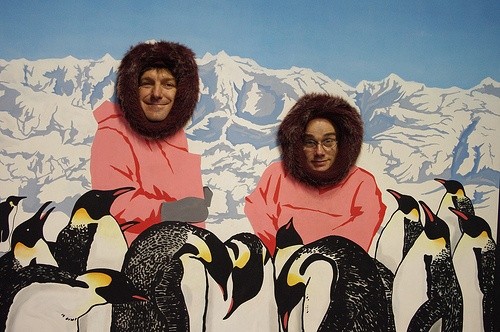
[302, 138, 339, 151]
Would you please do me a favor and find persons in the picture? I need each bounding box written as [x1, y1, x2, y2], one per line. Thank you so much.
[302, 115, 338, 171]
[138, 62, 178, 122]
